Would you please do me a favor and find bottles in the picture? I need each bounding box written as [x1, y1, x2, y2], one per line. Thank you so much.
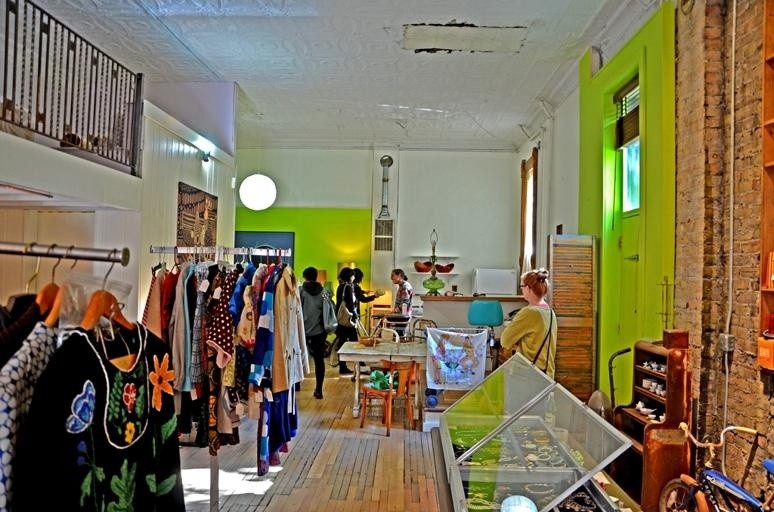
[402, 302, 407, 316]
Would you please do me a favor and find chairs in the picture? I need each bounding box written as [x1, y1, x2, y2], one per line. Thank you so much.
[359, 319, 440, 436]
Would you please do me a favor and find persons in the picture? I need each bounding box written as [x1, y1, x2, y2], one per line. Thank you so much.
[500, 268, 557, 421]
[458, 335, 480, 376]
[335, 266, 370, 376]
[293, 267, 330, 399]
[431, 335, 451, 384]
[351, 267, 379, 322]
[391, 268, 414, 340]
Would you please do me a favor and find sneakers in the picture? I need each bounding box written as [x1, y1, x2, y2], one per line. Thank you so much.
[339, 368, 353, 374]
[314, 389, 322, 399]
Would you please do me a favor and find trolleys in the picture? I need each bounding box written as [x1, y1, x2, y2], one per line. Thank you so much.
[424, 326, 488, 410]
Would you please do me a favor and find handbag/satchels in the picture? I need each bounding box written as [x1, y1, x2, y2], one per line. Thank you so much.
[336, 300, 355, 328]
[322, 293, 338, 332]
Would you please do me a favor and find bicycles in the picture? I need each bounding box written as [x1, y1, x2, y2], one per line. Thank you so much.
[658, 419, 774, 512]
[327, 309, 400, 368]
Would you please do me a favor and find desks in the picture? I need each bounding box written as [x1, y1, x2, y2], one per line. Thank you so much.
[336, 341, 431, 418]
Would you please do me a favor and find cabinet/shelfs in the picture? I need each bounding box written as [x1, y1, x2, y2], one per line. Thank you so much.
[612, 341, 693, 512]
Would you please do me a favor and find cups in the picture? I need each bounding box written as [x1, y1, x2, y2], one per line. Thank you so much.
[648, 414, 656, 419]
[643, 361, 666, 373]
[642, 378, 666, 397]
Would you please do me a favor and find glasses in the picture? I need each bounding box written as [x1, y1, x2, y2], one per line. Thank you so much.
[519, 285, 526, 288]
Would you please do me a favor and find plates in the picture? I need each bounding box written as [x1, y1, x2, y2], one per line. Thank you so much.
[639, 408, 657, 416]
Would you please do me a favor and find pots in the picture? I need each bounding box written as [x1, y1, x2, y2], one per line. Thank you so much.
[373, 315, 410, 322]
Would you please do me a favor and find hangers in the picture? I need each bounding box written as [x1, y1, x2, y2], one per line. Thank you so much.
[144, 245, 295, 296]
[0, 240, 178, 401]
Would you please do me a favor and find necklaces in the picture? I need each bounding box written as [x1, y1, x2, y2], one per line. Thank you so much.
[465, 481, 597, 512]
